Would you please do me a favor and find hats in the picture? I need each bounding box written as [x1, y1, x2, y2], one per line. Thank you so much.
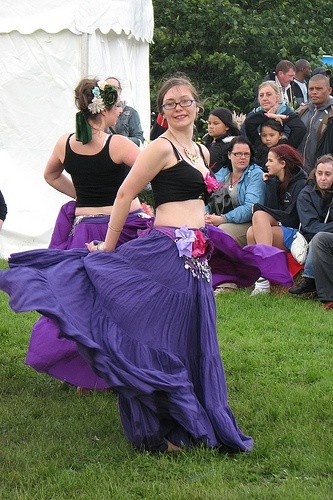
[311, 67, 332, 77]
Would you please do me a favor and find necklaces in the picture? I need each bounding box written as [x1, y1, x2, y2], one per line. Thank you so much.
[169, 130, 199, 166]
[230, 172, 242, 188]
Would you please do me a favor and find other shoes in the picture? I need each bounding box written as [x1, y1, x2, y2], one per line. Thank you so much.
[288, 278, 316, 294]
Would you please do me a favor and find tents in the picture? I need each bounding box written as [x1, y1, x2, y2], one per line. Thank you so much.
[0, 0, 154, 260]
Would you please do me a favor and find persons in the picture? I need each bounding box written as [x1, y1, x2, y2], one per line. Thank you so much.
[149, 59, 333, 312]
[0, 73, 255, 460]
[0, 190, 8, 232]
[25, 76, 155, 391]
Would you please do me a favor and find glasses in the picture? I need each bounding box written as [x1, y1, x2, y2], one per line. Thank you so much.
[161, 99, 196, 110]
[231, 152, 250, 157]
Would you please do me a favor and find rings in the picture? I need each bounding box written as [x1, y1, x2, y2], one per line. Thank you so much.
[210, 220, 212, 223]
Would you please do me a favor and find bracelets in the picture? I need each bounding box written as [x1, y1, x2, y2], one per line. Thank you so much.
[97, 241, 114, 253]
[107, 223, 124, 233]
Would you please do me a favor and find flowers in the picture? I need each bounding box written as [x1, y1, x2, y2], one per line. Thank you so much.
[205, 173, 223, 193]
[142, 203, 154, 216]
[175, 226, 209, 257]
[88, 80, 118, 116]
